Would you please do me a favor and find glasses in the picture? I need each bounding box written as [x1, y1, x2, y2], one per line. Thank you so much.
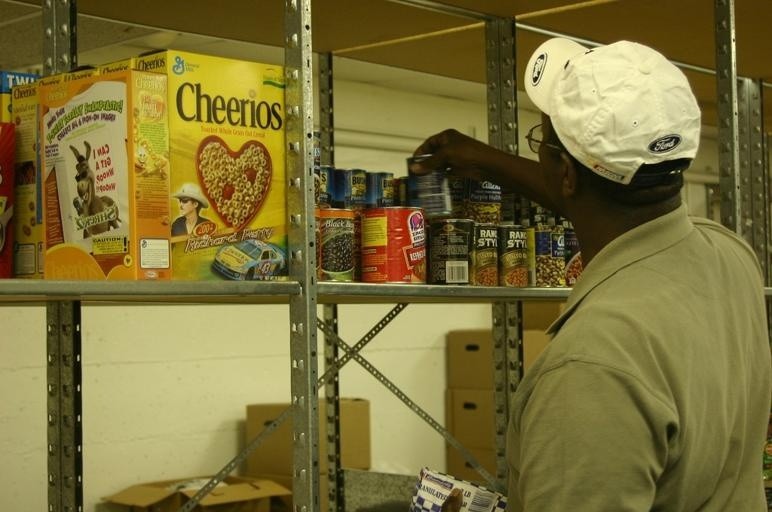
[525, 124, 582, 167]
[179, 198, 188, 203]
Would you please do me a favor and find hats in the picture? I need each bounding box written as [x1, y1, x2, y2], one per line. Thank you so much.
[524, 38, 701, 187]
[174, 184, 209, 208]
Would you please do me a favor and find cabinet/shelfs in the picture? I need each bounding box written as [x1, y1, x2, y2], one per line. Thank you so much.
[0, 0, 772, 512]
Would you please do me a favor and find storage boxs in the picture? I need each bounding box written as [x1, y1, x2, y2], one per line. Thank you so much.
[100, 475, 292, 512]
[0, 49, 290, 280]
[246, 397, 371, 475]
[446, 299, 573, 484]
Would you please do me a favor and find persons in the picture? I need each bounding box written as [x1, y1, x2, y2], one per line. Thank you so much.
[407, 37, 772, 512]
[20, 160, 36, 186]
[169, 182, 212, 238]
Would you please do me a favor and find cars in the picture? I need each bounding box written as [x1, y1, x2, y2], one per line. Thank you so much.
[213, 239, 285, 280]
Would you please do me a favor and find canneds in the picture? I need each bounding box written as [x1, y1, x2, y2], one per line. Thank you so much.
[321, 210, 354, 281]
[405, 154, 445, 213]
[313, 208, 324, 280]
[362, 207, 426, 284]
[426, 218, 472, 284]
[499, 225, 529, 286]
[531, 200, 575, 229]
[499, 184, 530, 221]
[350, 209, 364, 282]
[393, 176, 409, 206]
[448, 171, 471, 215]
[466, 168, 500, 223]
[536, 226, 566, 287]
[319, 165, 337, 209]
[565, 231, 583, 287]
[338, 169, 367, 207]
[367, 172, 394, 206]
[469, 222, 499, 285]
